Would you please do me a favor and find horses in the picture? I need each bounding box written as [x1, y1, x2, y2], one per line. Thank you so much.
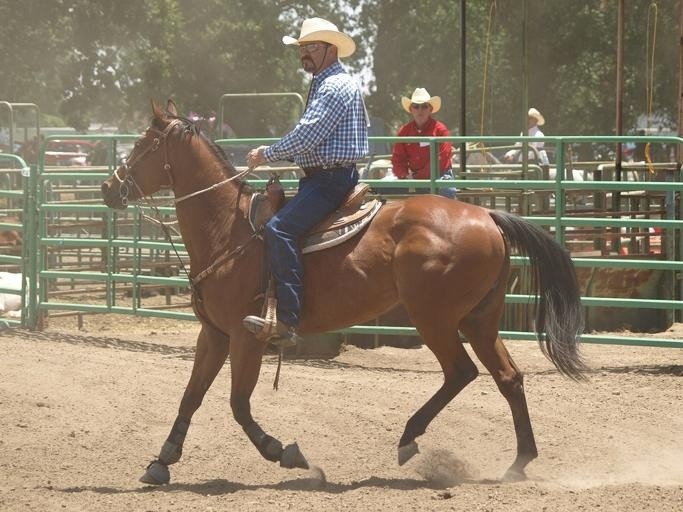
[452, 137, 597, 182]
[100, 97, 602, 486]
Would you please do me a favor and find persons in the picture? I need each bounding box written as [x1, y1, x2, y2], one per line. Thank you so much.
[505, 108, 549, 166]
[391, 87, 456, 198]
[66, 145, 87, 166]
[242, 18, 370, 348]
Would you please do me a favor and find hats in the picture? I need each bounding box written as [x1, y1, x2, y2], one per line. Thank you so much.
[401, 88, 441, 114]
[528, 108, 545, 126]
[282, 17, 356, 58]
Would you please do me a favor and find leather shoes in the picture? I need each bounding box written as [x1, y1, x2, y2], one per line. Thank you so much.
[242, 315, 297, 349]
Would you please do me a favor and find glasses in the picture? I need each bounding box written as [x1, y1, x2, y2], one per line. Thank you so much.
[411, 103, 428, 108]
[297, 44, 328, 56]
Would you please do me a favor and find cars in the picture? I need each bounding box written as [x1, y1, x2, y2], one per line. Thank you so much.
[0, 123, 136, 167]
